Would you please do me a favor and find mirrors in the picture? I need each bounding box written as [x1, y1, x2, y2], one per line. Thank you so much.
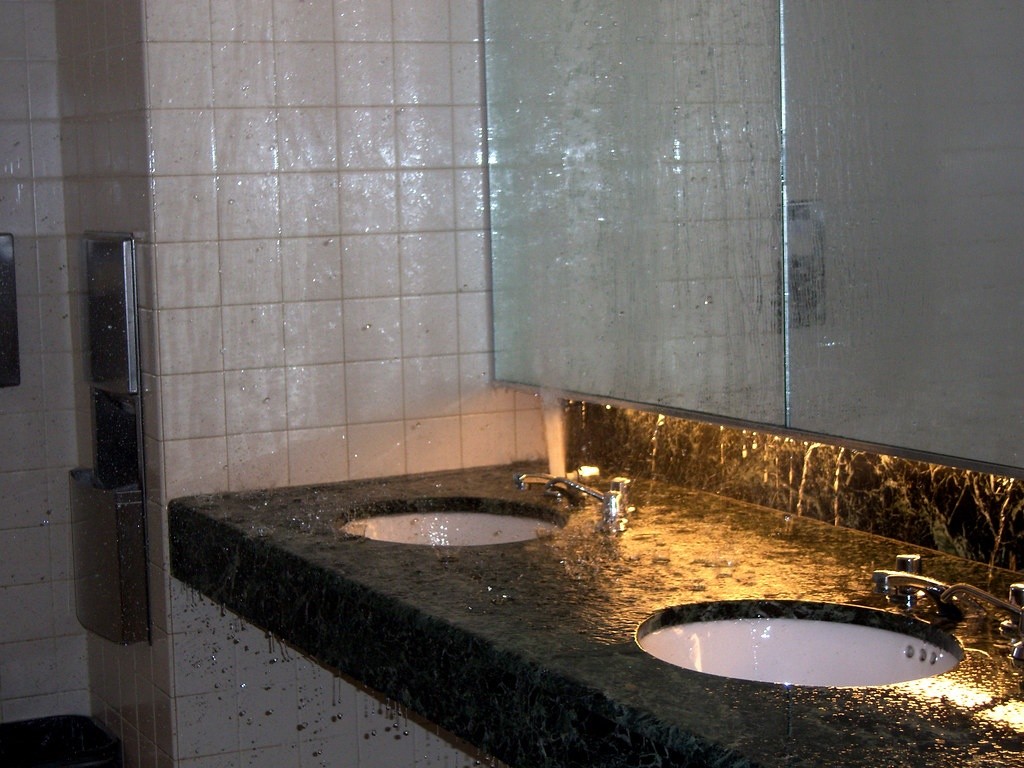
[482, 0, 1024, 480]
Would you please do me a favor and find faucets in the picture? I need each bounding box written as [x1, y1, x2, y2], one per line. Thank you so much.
[550, 476, 627, 533]
[941, 580, 1023, 657]
[875, 568, 989, 622]
[516, 474, 592, 509]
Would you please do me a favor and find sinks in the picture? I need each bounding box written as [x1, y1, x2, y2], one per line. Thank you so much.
[335, 490, 568, 549]
[634, 595, 966, 690]
